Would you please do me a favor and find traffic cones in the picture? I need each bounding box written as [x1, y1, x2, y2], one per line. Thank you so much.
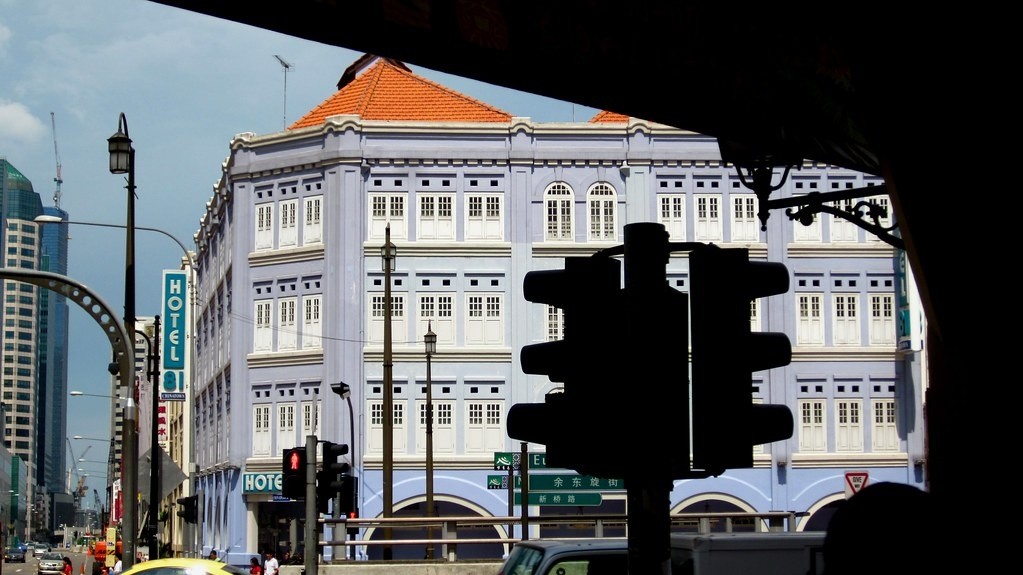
[80, 562, 85, 575]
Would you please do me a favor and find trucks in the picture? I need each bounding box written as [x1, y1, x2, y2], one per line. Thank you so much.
[494, 532, 830, 575]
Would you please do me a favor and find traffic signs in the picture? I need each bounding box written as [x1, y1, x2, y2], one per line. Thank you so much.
[527, 475, 624, 490]
[512, 493, 602, 506]
[528, 452, 551, 470]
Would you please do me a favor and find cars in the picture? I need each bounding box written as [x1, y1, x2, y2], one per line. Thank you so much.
[113, 557, 249, 575]
[4, 548, 25, 563]
[15, 540, 37, 552]
[36, 552, 65, 575]
[32, 543, 50, 558]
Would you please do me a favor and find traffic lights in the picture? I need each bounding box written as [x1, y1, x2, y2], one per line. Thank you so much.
[73, 531, 77, 538]
[282, 448, 307, 500]
[506, 255, 622, 450]
[176, 495, 199, 525]
[339, 475, 359, 514]
[323, 440, 350, 498]
[692, 242, 793, 474]
[345, 510, 358, 535]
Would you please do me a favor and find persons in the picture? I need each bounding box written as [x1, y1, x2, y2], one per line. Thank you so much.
[59, 556, 73, 575]
[209, 551, 222, 563]
[109, 552, 122, 572]
[249, 557, 261, 575]
[264, 552, 278, 575]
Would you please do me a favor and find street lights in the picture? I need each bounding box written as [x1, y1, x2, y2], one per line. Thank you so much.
[330, 381, 358, 560]
[33, 214, 195, 559]
[379, 224, 398, 564]
[421, 321, 439, 560]
[107, 111, 138, 567]
